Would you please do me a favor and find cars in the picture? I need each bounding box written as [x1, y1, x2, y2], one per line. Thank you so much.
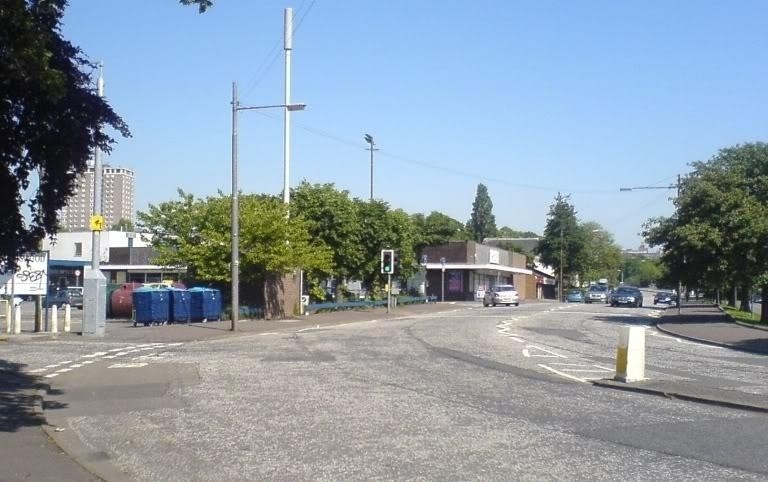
[653, 292, 672, 305]
[483, 285, 520, 307]
[41, 287, 83, 310]
[567, 279, 643, 307]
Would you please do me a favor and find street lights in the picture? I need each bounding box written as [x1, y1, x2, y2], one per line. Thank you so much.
[617, 269, 623, 283]
[619, 174, 681, 307]
[231, 81, 307, 332]
[363, 133, 379, 203]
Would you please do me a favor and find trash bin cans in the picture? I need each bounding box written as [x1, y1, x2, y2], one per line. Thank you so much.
[132, 287, 222, 326]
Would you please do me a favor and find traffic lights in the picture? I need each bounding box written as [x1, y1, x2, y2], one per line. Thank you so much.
[380, 250, 394, 274]
